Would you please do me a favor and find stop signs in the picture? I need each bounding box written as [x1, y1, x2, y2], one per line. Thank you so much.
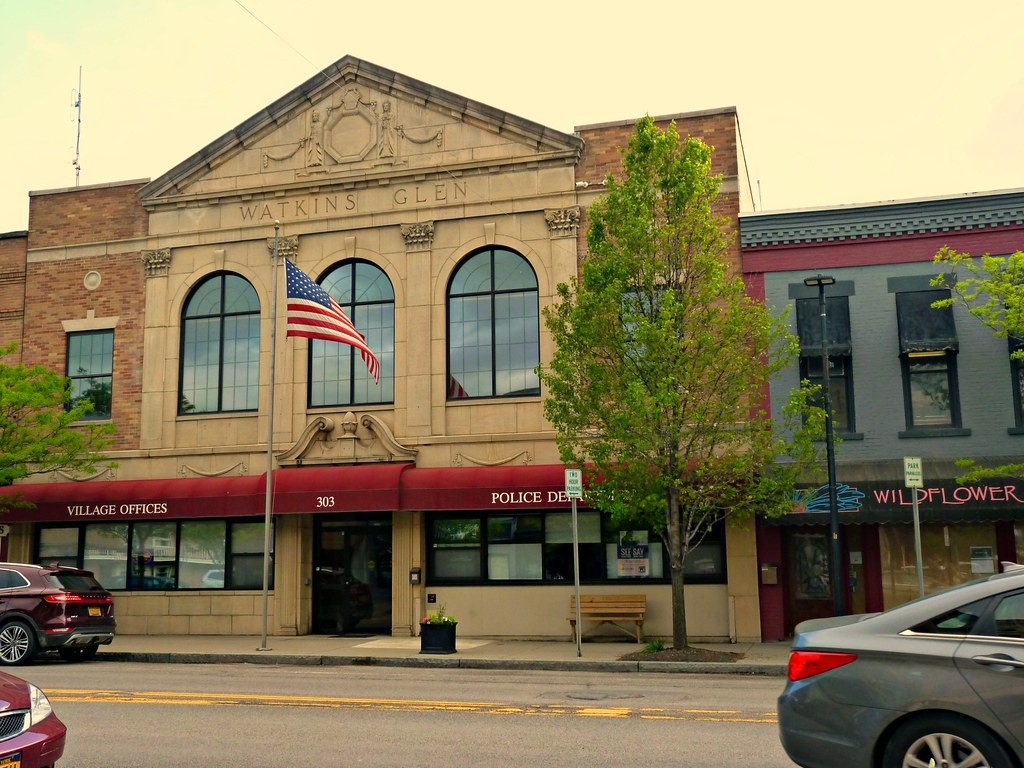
[143, 551, 151, 562]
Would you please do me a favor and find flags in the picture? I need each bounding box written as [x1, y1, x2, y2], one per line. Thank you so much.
[449, 375, 469, 398]
[283, 258, 380, 385]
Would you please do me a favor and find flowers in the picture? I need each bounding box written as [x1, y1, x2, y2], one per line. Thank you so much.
[420, 601, 456, 626]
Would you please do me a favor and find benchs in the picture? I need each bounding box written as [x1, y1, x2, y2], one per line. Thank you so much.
[566, 594, 647, 645]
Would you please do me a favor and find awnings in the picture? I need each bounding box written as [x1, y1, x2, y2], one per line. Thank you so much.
[0, 461, 742, 524]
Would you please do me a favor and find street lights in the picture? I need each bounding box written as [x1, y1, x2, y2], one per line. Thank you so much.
[805, 273, 846, 616]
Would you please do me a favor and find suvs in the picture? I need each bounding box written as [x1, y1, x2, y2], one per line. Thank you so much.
[202, 569, 226, 588]
[0, 562, 116, 665]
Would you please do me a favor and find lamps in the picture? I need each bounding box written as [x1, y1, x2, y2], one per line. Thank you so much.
[575, 181, 589, 189]
[598, 180, 608, 186]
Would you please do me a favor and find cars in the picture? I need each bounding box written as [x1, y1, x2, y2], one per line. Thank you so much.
[882, 560, 1024, 608]
[1, 671, 65, 768]
[777, 560, 1024, 768]
[108, 574, 175, 590]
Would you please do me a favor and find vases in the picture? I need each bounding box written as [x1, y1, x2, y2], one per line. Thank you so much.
[419, 622, 458, 656]
